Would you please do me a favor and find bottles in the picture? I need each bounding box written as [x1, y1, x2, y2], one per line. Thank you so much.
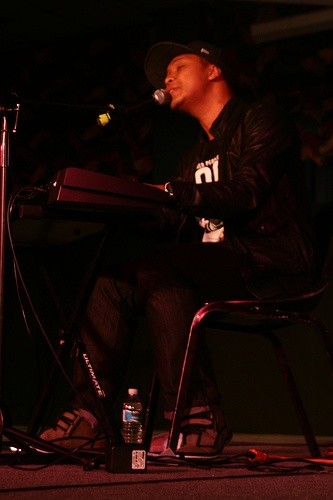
[122, 389, 143, 444]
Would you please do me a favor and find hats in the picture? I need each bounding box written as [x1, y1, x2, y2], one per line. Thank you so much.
[144, 40, 224, 90]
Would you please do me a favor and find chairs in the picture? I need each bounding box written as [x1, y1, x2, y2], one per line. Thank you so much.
[137, 157, 332, 456]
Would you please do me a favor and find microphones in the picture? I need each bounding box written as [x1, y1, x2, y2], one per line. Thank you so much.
[110, 88, 170, 119]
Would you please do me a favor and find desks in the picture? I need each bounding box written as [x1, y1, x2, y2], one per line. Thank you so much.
[3, 201, 147, 471]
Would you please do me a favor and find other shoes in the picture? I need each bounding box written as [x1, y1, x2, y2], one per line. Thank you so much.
[38, 407, 99, 451]
[148, 407, 218, 455]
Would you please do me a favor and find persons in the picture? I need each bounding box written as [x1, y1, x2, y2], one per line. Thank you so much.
[29, 38, 310, 466]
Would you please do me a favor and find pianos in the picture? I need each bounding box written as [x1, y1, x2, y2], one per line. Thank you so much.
[0, 166, 167, 475]
